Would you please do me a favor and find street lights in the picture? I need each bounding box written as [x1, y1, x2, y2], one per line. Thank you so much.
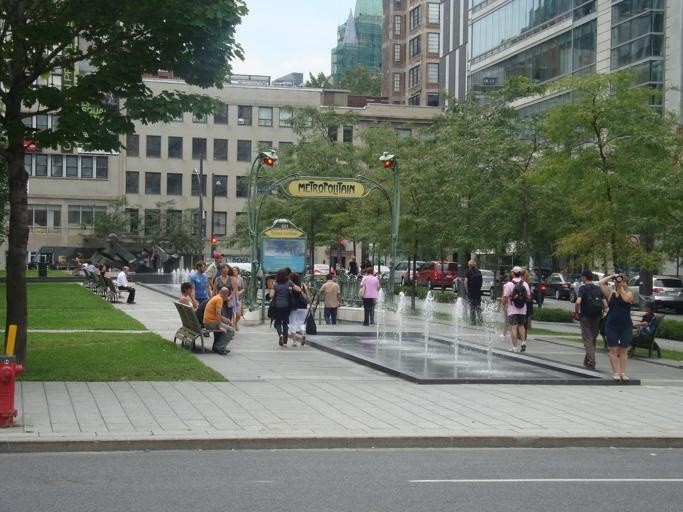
[248, 148, 280, 312]
[209, 180, 222, 258]
[378, 150, 401, 311]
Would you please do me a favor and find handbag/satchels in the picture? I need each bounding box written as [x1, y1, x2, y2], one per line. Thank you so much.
[584, 297, 603, 315]
[289, 295, 298, 311]
[306, 314, 316, 333]
[358, 286, 365, 298]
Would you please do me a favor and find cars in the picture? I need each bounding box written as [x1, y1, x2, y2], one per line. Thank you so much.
[451, 268, 495, 295]
[490, 265, 683, 314]
[357, 265, 391, 281]
[306, 263, 337, 276]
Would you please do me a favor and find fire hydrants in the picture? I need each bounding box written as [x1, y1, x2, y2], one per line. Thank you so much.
[1, 354, 25, 428]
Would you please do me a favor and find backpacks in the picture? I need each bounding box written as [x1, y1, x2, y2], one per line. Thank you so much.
[511, 280, 527, 308]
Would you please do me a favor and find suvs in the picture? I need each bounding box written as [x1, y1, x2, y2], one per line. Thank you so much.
[382, 259, 425, 287]
[415, 260, 460, 291]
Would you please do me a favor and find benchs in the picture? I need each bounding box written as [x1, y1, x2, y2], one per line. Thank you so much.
[83, 268, 129, 302]
[600, 308, 668, 359]
[173, 301, 223, 352]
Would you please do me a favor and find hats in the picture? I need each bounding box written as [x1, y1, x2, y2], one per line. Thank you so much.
[511, 266, 521, 272]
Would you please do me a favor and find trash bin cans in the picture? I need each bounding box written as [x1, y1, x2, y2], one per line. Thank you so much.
[37, 262, 48, 276]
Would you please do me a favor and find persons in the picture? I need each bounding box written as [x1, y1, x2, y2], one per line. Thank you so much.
[575, 270, 656, 380]
[268, 267, 342, 347]
[361, 267, 379, 325]
[464, 259, 484, 325]
[348, 256, 371, 276]
[75, 254, 136, 304]
[180, 254, 245, 355]
[500, 266, 533, 352]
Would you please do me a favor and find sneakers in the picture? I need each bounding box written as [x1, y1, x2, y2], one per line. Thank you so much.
[214, 348, 229, 354]
[510, 348, 519, 353]
[520, 342, 526, 351]
[279, 334, 305, 347]
[613, 372, 628, 380]
[203, 332, 209, 337]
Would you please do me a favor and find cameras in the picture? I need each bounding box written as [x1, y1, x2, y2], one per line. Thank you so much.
[616, 276, 623, 282]
